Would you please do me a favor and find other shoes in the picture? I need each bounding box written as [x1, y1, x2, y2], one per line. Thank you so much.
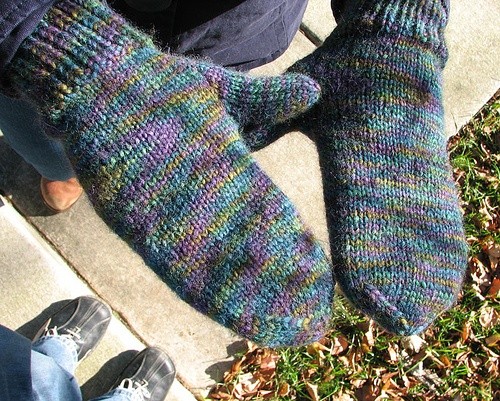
[39, 170, 83, 213]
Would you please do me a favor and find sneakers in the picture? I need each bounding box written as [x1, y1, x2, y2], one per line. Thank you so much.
[31, 295, 112, 366]
[107, 347, 176, 401]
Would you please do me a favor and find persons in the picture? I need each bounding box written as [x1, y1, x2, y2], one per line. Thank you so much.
[0, 0, 466, 349]
[0, 295, 177, 401]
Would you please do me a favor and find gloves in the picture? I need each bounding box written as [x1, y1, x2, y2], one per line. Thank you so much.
[2, 0, 335, 346]
[237, 0, 467, 336]
